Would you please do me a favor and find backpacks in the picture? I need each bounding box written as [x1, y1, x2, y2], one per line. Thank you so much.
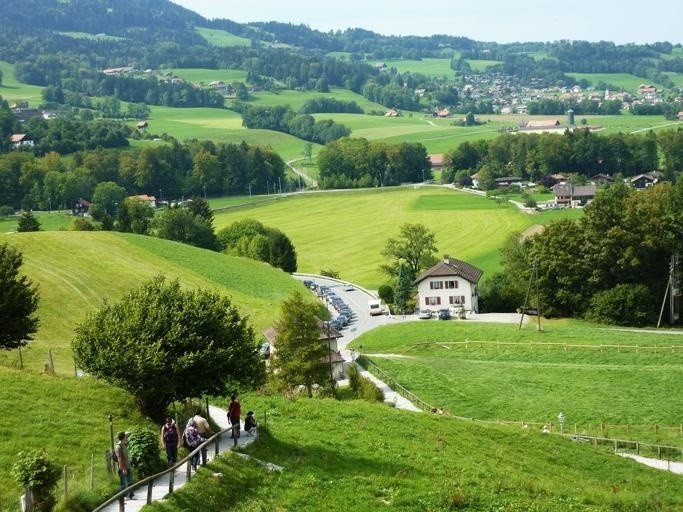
[163, 427, 176, 443]
[186, 428, 198, 447]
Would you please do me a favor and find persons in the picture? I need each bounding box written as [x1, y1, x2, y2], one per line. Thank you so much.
[244, 410, 257, 437]
[160, 417, 180, 469]
[114, 432, 137, 503]
[180, 420, 204, 470]
[185, 407, 212, 462]
[227, 394, 241, 439]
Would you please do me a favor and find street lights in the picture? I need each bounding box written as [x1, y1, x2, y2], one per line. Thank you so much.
[108, 413, 116, 476]
[421, 169, 424, 182]
[558, 412, 566, 436]
[202, 184, 207, 202]
[248, 170, 303, 198]
[159, 188, 163, 202]
[114, 203, 120, 219]
[379, 173, 383, 187]
[48, 197, 52, 213]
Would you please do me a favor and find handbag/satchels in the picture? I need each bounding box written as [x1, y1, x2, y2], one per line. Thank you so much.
[112, 444, 123, 462]
[180, 434, 186, 449]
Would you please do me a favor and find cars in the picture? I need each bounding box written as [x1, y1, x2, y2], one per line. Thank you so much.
[418, 308, 433, 319]
[431, 179, 436, 183]
[302, 279, 353, 332]
[436, 308, 450, 320]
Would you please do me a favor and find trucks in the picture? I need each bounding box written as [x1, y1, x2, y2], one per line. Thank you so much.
[368, 299, 383, 316]
[259, 341, 271, 359]
